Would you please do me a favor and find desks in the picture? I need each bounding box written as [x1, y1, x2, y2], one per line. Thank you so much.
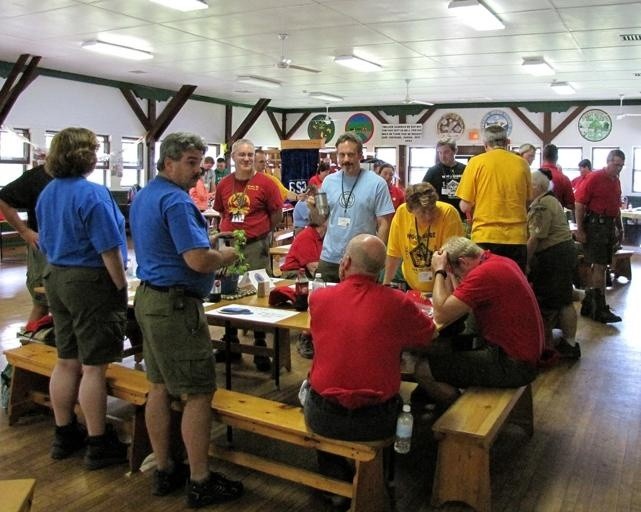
[30, 279, 468, 371]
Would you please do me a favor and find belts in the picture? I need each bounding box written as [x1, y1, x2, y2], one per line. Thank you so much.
[311, 393, 399, 415]
[140, 282, 167, 293]
[225, 232, 265, 245]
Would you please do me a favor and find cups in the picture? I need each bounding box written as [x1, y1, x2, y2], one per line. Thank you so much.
[313, 192, 327, 216]
[217, 234, 234, 250]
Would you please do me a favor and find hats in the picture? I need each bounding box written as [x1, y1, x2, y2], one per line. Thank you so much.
[268, 287, 296, 308]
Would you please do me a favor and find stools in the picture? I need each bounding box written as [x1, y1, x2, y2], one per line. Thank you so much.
[611, 251, 633, 280]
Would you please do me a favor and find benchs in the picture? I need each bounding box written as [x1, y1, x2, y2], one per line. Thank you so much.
[0, 341, 534, 511]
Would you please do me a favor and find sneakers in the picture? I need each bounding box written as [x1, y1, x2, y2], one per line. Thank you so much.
[51, 437, 129, 467]
[212, 335, 313, 372]
[555, 336, 581, 359]
[149, 462, 243, 507]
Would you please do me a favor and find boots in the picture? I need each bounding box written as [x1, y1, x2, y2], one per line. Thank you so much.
[580, 288, 622, 322]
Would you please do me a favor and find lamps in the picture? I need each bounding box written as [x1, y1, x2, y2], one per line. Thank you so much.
[81, 0, 581, 103]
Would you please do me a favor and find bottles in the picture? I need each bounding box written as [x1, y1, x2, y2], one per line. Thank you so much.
[312, 273, 326, 290]
[208, 273, 221, 303]
[392, 404, 414, 455]
[293, 267, 308, 312]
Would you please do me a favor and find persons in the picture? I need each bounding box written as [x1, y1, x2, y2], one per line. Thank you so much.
[0, 126, 625, 512]
